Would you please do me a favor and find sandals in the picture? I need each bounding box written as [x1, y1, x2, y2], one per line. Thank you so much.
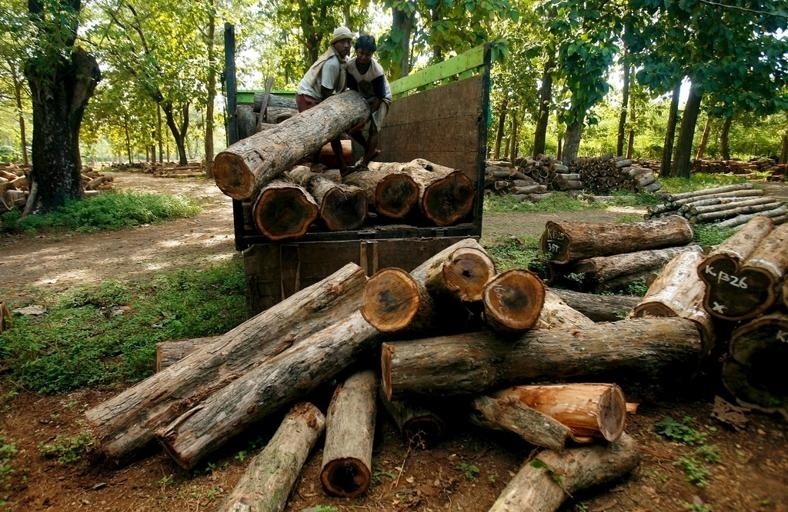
[340, 149, 381, 178]
[310, 165, 325, 173]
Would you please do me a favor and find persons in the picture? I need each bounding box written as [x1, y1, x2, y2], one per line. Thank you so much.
[297, 26, 359, 178]
[347, 33, 393, 169]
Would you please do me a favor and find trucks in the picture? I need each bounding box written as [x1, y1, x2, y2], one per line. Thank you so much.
[225, 42, 492, 310]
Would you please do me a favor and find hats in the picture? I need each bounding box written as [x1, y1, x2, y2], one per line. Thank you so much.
[329, 26, 356, 44]
